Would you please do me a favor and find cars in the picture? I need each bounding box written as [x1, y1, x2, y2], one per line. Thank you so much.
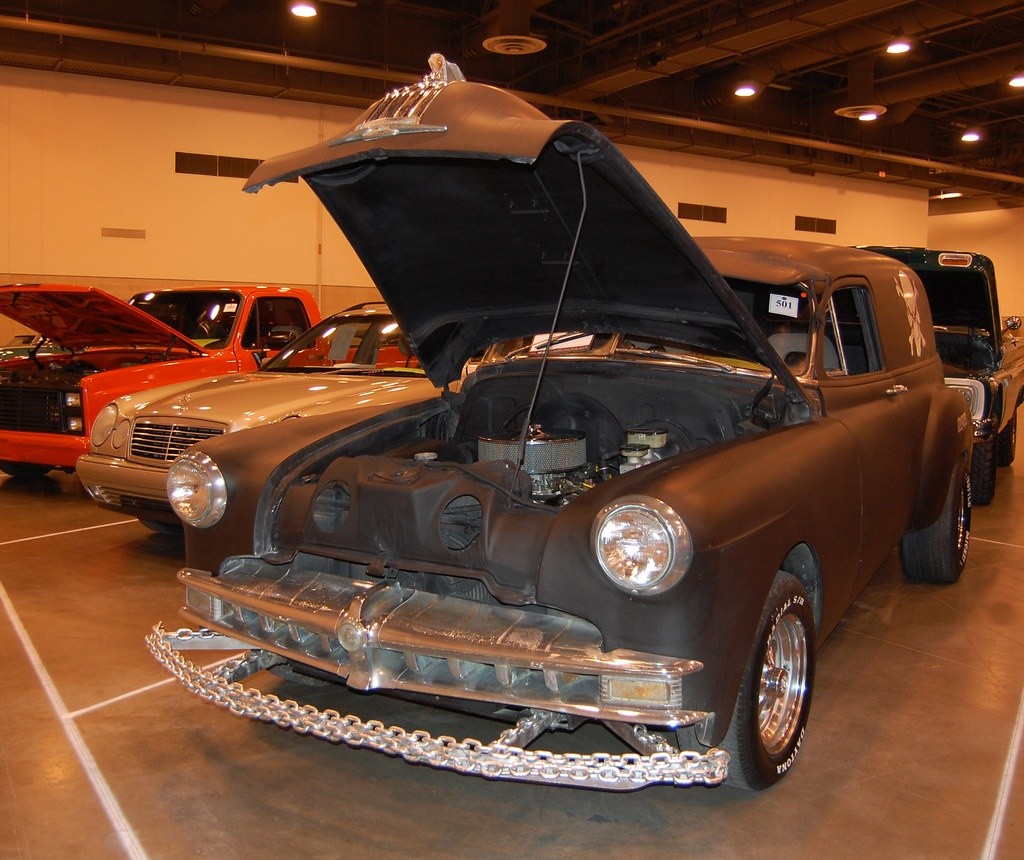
[828, 241, 1024, 510]
[78, 300, 534, 540]
[0, 281, 420, 483]
[144, 54, 976, 794]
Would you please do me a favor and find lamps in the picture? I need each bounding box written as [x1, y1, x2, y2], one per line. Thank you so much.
[1009, 64, 1024, 87]
[960, 121, 980, 142]
[885, 27, 910, 54]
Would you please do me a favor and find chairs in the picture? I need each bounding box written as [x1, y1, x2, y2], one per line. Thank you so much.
[271, 325, 302, 339]
[769, 331, 841, 374]
